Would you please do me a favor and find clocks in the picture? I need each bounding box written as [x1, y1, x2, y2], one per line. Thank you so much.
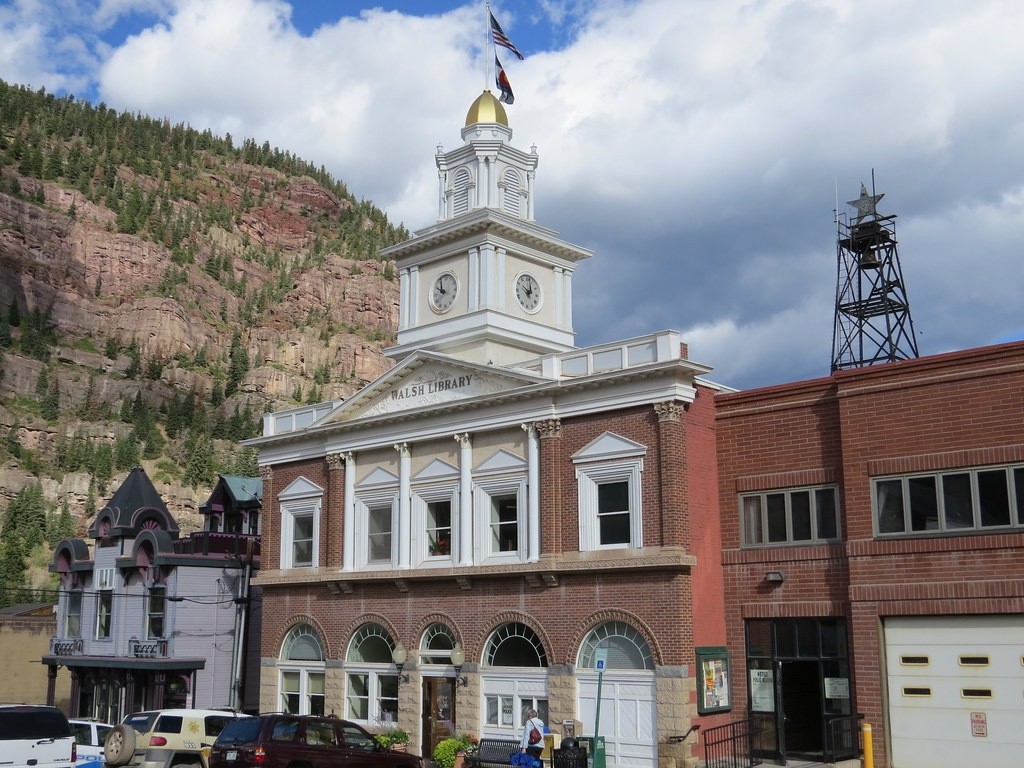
[428, 269, 461, 315]
[512, 269, 544, 315]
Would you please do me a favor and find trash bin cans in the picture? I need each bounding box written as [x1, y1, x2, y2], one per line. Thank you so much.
[553, 736, 588, 768]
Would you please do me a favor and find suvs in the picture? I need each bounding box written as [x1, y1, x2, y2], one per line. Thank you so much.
[208, 711, 426, 768]
[104, 708, 256, 768]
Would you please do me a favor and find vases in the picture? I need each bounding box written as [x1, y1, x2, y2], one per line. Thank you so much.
[432, 551, 445, 555]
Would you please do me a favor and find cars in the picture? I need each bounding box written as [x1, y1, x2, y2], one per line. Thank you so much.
[68, 717, 114, 768]
[0, 701, 77, 768]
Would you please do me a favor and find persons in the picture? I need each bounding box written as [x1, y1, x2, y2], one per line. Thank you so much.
[521, 709, 545, 768]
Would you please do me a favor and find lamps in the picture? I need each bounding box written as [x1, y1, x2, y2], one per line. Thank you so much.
[391, 639, 410, 684]
[450, 639, 469, 689]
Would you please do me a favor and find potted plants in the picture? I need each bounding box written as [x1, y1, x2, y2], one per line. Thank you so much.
[433, 738, 470, 768]
[366, 727, 410, 752]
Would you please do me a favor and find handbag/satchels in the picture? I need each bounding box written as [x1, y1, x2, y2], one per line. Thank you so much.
[527, 719, 542, 745]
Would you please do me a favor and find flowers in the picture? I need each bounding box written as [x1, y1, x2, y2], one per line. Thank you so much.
[462, 733, 479, 746]
[429, 538, 450, 553]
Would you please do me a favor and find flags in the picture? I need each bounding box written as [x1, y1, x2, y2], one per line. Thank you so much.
[489, 8, 524, 59]
[494, 53, 514, 105]
